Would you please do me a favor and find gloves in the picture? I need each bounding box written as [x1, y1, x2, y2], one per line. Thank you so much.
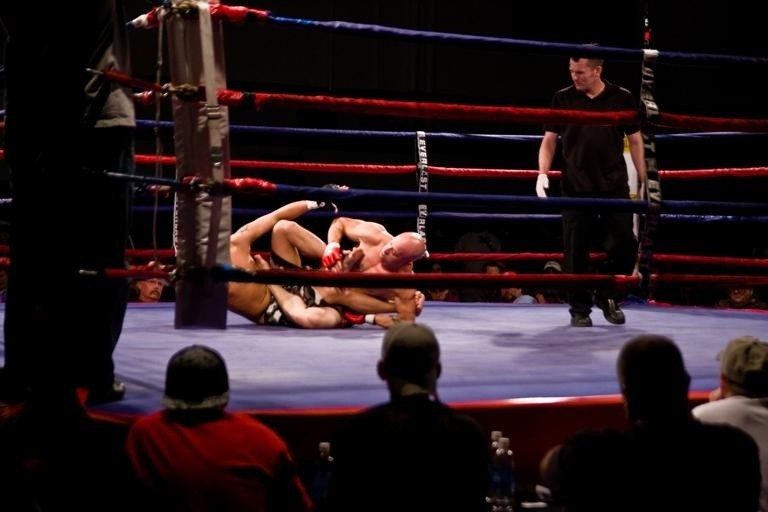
[536, 174, 549, 197]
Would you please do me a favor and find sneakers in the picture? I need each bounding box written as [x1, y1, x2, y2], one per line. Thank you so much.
[595, 298, 625, 324]
[571, 313, 592, 327]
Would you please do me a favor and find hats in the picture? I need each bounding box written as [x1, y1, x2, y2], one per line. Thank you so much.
[716, 335, 768, 387]
[160, 344, 229, 408]
[382, 323, 437, 373]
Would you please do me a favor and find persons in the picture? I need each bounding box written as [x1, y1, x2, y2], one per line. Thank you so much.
[252, 216, 430, 327]
[711, 281, 768, 311]
[420, 257, 564, 306]
[534, 43, 646, 328]
[546, 332, 763, 510]
[78, 46, 137, 402]
[305, 323, 493, 512]
[690, 334, 768, 510]
[230, 199, 425, 329]
[133, 276, 167, 303]
[125, 343, 312, 512]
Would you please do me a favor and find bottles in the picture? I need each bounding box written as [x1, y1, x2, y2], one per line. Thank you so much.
[488, 432, 518, 510]
[314, 441, 335, 499]
